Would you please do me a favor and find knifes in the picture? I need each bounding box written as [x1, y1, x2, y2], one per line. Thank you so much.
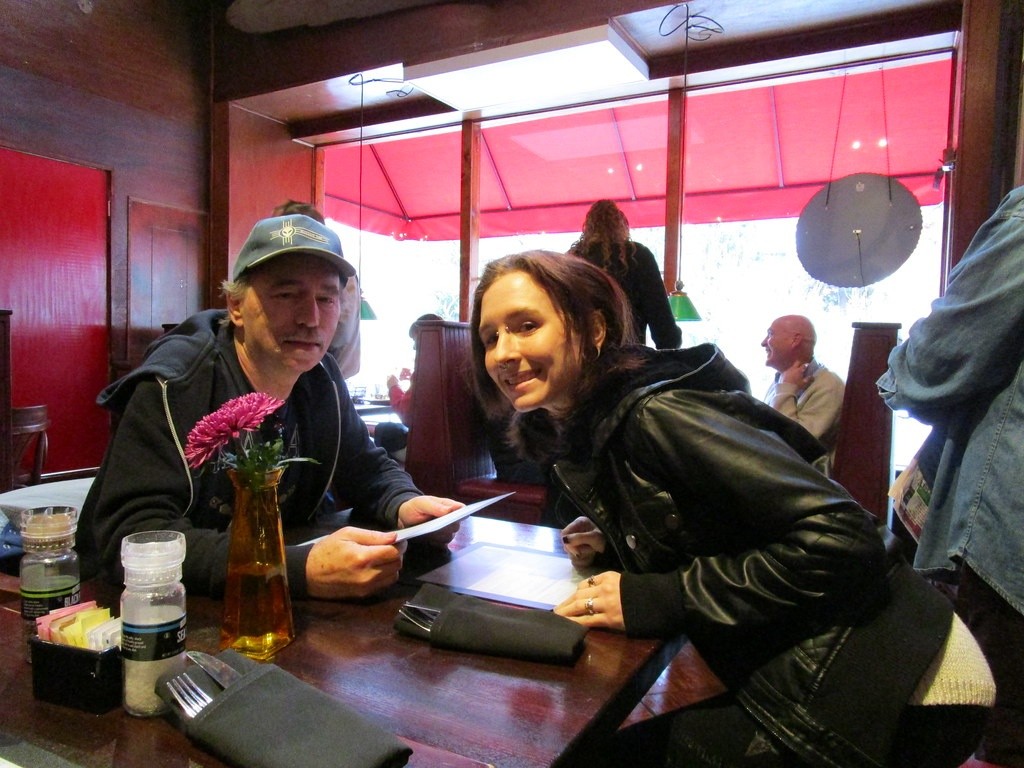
[403, 604, 441, 613]
[186, 651, 242, 689]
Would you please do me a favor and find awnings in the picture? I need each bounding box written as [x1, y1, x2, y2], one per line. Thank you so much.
[326, 36, 965, 333]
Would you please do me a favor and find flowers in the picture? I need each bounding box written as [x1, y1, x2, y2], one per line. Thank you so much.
[183, 390, 323, 641]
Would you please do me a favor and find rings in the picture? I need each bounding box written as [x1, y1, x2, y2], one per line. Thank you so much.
[585, 597, 597, 614]
[586, 575, 596, 586]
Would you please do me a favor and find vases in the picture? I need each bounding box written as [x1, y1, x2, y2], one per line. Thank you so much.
[218, 468, 296, 660]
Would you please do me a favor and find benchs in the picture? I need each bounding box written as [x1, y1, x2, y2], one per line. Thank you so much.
[404, 320, 552, 526]
[831, 322, 906, 532]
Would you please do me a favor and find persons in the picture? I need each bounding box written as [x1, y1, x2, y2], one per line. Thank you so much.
[874, 184, 1024, 768]
[367, 311, 444, 460]
[272, 199, 361, 382]
[564, 196, 683, 353]
[759, 313, 847, 445]
[74, 210, 466, 605]
[469, 255, 997, 768]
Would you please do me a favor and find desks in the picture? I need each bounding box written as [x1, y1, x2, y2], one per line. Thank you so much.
[0, 516, 692, 768]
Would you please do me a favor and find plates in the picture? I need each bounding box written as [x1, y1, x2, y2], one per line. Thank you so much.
[365, 399, 391, 405]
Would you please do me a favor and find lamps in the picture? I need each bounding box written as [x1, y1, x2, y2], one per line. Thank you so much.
[356, 75, 378, 320]
[667, 4, 703, 322]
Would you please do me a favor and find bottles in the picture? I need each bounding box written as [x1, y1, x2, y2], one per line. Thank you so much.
[19, 506, 79, 664]
[120, 530, 186, 717]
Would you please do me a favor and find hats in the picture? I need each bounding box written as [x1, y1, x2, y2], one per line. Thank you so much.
[232, 214, 355, 289]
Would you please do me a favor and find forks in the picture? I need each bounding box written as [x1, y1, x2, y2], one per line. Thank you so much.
[399, 601, 436, 632]
[166, 673, 213, 719]
